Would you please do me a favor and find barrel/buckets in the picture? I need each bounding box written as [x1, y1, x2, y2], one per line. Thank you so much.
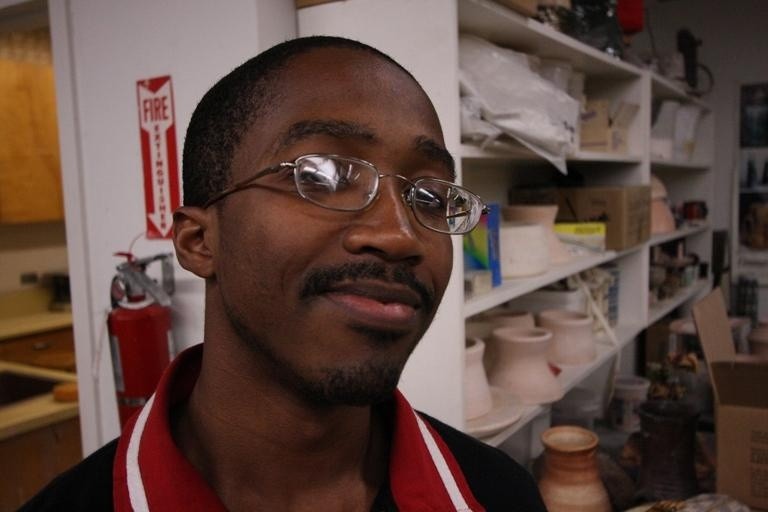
[609, 376, 650, 432]
[548, 389, 601, 430]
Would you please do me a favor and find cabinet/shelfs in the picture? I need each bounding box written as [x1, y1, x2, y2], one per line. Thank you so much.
[1, 280, 81, 511]
[650, 78, 735, 466]
[296, 0, 646, 470]
[736, 83, 766, 342]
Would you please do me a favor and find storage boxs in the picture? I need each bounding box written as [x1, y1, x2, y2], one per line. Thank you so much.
[579, 98, 640, 155]
[692, 287, 768, 512]
[512, 185, 649, 251]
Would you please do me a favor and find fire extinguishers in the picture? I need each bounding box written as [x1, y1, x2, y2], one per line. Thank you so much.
[105, 251, 176, 436]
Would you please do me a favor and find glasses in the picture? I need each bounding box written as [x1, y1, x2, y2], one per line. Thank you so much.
[202, 150, 492, 237]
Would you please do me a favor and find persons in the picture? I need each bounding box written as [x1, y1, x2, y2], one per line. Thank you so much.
[6, 38, 550, 512]
